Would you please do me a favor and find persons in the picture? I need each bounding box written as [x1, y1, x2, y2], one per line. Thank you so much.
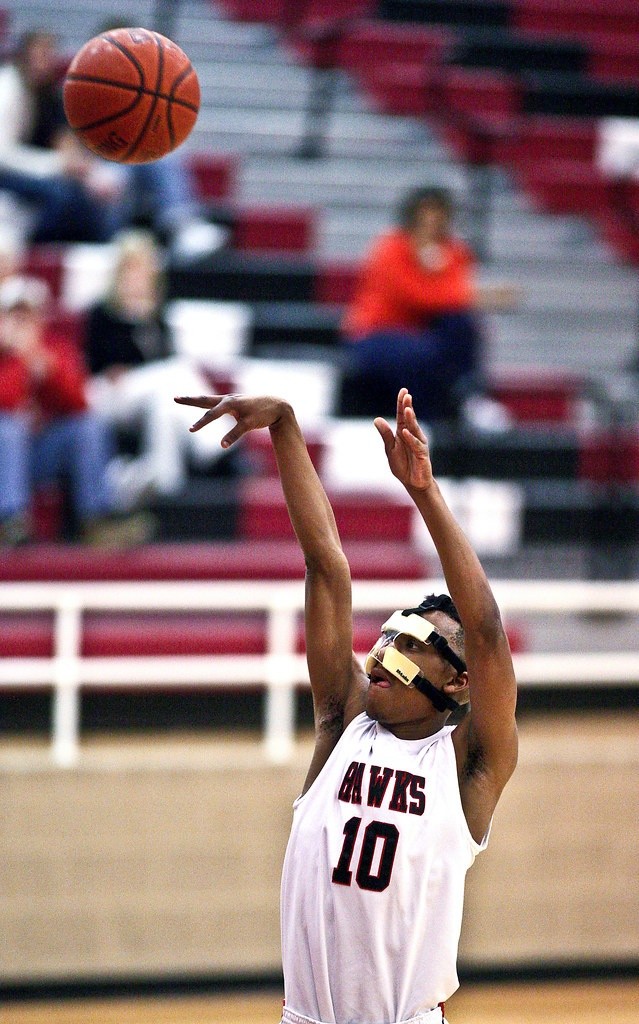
[411, 382, 519, 556]
[331, 186, 518, 443]
[174, 387, 519, 1024]
[0, 8, 252, 549]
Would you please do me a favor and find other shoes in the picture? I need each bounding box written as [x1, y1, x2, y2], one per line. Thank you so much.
[83, 513, 153, 548]
[0, 515, 37, 545]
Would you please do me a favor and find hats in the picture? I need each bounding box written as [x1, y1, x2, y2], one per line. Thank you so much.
[0, 277, 45, 312]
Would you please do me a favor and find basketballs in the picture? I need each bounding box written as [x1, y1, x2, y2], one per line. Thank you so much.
[63, 27, 201, 162]
